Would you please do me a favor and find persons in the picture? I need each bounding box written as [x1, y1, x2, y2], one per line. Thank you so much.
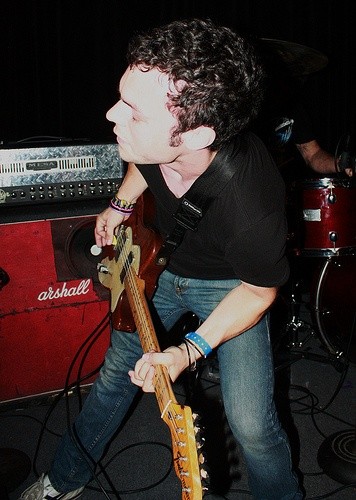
[253, 111, 353, 183]
[17, 17, 308, 500]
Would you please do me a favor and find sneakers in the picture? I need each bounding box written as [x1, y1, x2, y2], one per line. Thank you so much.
[18, 473, 85, 500]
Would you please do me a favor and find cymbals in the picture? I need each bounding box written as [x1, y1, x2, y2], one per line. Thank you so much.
[255, 38, 328, 79]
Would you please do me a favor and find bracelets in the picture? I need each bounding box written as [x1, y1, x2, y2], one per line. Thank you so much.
[178, 331, 213, 373]
[108, 195, 138, 215]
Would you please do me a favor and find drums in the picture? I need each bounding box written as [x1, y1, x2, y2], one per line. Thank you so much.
[290, 178, 356, 253]
[276, 253, 356, 365]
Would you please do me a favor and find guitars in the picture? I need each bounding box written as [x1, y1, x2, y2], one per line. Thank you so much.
[91, 191, 210, 500]
